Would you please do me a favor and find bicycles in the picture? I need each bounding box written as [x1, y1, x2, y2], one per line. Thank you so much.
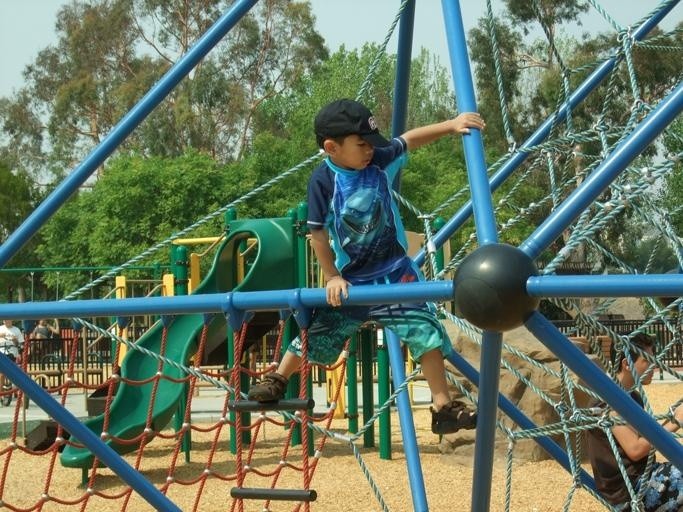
[0, 341, 29, 409]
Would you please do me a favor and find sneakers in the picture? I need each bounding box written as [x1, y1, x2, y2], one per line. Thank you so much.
[248, 373, 289, 402]
[430, 400, 477, 435]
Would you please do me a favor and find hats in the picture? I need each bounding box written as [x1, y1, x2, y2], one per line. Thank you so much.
[314, 99, 393, 148]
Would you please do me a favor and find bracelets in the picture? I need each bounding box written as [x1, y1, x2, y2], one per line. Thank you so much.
[669, 416, 680, 428]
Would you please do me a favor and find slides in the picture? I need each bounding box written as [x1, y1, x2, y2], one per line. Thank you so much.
[60, 219, 296, 469]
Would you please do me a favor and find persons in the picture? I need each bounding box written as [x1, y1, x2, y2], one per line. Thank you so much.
[243, 94, 488, 436]
[28, 319, 59, 353]
[584, 328, 682, 511]
[0, 319, 24, 402]
